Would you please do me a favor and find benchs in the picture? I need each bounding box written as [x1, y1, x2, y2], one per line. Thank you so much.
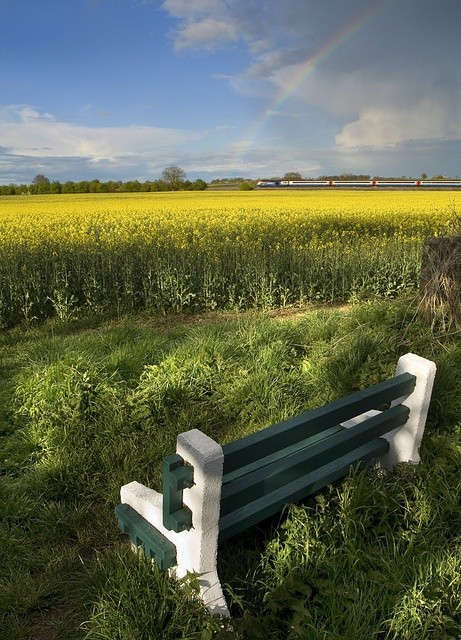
[119, 354, 438, 619]
